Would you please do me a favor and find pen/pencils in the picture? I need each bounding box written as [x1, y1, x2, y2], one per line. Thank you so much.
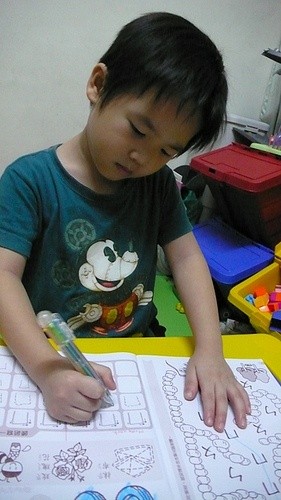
[35, 310, 115, 406]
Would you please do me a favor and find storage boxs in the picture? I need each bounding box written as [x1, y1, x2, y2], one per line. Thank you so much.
[188, 219, 274, 319]
[227, 242, 281, 333]
[188, 143, 281, 248]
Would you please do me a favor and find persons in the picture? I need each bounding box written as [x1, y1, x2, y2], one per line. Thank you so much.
[0, 11, 252, 434]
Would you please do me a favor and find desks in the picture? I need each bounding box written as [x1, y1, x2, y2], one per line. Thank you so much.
[0, 333, 281, 500]
